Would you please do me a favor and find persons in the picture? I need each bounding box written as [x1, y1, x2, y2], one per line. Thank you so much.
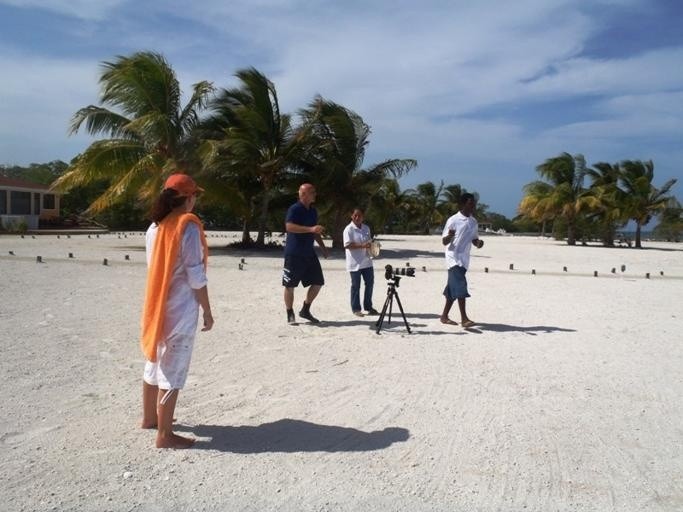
[342, 207, 378, 317]
[441, 193, 484, 328]
[282, 183, 329, 323]
[139, 173, 213, 450]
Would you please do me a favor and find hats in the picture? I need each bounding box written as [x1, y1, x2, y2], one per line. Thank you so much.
[162, 173, 205, 198]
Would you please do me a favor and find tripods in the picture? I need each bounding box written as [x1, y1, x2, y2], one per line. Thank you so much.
[376, 283, 412, 335]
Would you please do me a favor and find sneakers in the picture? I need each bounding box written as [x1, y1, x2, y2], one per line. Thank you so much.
[287, 311, 296, 323]
[366, 307, 379, 316]
[299, 310, 320, 324]
[352, 310, 365, 318]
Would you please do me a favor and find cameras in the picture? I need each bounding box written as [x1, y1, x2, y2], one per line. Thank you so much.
[385, 265, 415, 283]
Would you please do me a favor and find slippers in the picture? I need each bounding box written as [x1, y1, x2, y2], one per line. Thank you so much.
[439, 317, 459, 326]
[460, 320, 480, 328]
